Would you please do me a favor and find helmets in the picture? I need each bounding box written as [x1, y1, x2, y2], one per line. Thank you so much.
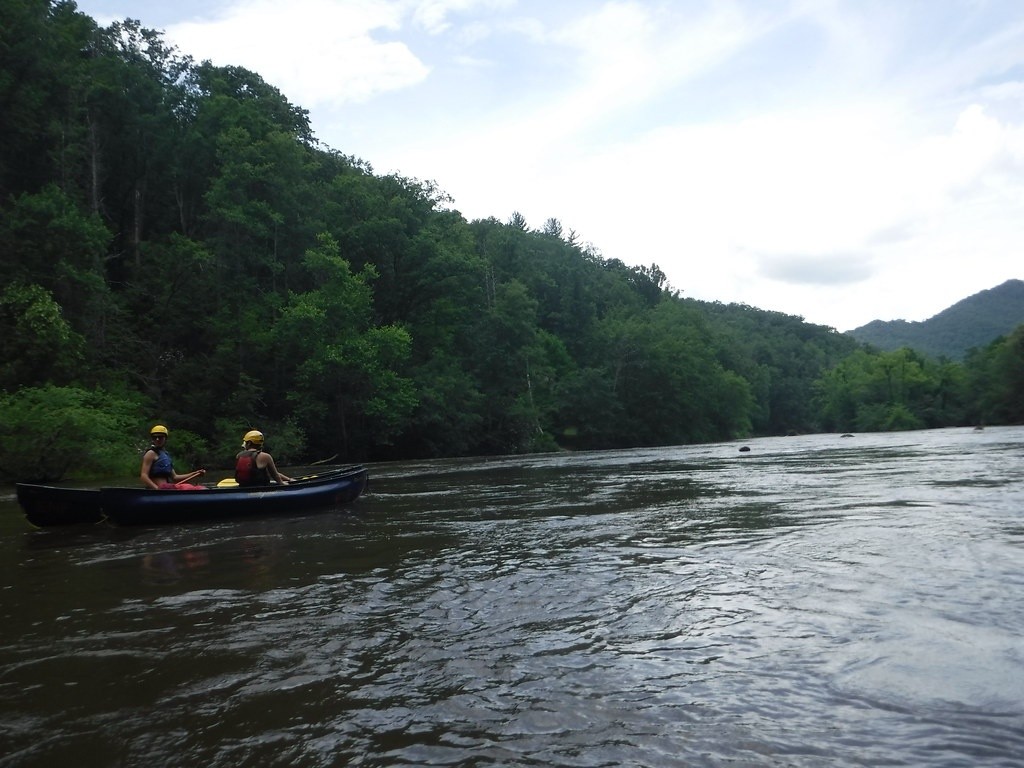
[150, 425, 168, 437]
[242, 430, 265, 445]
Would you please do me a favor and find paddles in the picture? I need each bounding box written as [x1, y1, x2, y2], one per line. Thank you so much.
[217, 478, 290, 486]
[175, 470, 203, 486]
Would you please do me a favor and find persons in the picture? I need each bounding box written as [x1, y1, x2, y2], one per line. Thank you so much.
[140, 425, 206, 489]
[234, 430, 297, 487]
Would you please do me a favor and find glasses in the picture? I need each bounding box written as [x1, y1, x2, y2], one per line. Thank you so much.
[151, 435, 164, 440]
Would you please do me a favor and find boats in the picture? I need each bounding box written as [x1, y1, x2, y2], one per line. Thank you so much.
[13, 464, 371, 529]
